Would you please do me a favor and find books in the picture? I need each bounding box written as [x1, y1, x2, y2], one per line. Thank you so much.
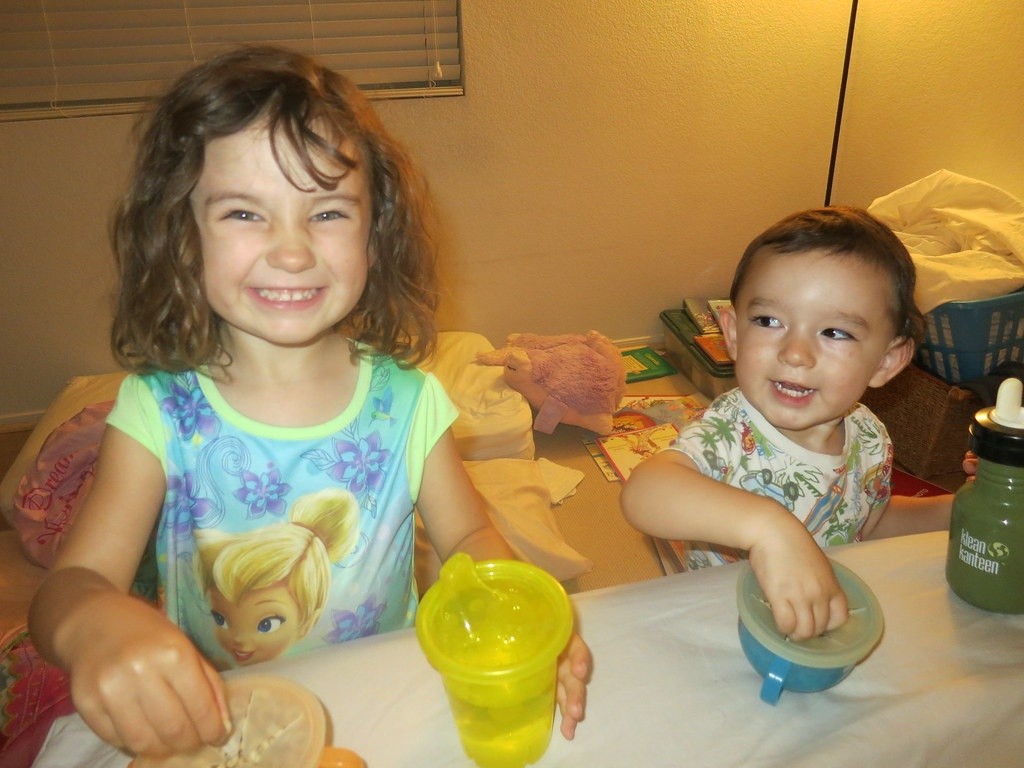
[592, 421, 680, 482]
[681, 295, 732, 364]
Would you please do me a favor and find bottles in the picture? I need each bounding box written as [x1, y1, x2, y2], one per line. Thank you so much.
[946, 378, 1024, 615]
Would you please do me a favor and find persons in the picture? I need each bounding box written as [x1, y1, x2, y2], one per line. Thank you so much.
[620, 205, 923, 636]
[24, 45, 592, 754]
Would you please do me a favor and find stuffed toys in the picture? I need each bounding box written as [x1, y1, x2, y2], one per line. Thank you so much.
[477, 331, 627, 434]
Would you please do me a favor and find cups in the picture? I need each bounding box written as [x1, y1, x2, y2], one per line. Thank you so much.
[415, 553, 574, 768]
[737, 557, 885, 706]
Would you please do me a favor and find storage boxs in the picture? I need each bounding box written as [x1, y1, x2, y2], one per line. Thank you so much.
[912, 289, 1024, 386]
[658, 309, 738, 401]
